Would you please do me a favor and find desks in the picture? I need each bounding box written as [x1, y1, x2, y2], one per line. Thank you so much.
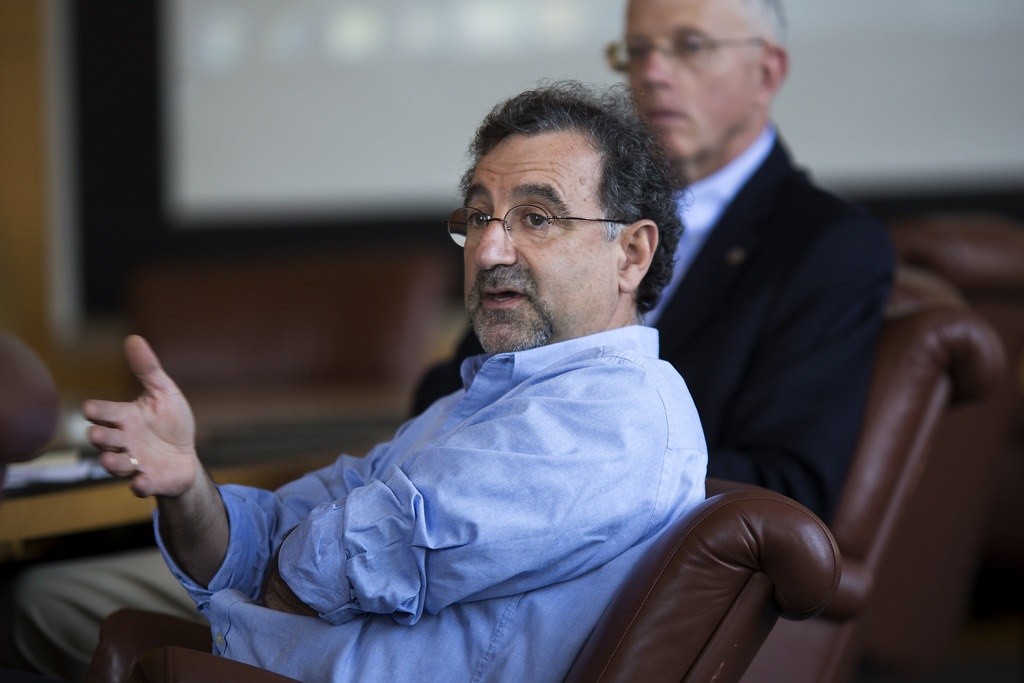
[0, 447, 277, 567]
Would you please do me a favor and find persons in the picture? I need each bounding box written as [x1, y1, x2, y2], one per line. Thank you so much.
[82, 79, 710, 683]
[0, 0, 897, 683]
[0, 331, 59, 464]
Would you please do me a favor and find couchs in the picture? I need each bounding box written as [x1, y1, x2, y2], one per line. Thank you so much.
[75, 221, 1024, 683]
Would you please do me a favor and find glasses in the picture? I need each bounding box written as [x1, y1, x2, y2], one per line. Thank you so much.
[607, 33, 768, 71]
[442, 204, 633, 247]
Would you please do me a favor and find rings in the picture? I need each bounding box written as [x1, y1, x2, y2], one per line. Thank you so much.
[129, 458, 140, 472]
